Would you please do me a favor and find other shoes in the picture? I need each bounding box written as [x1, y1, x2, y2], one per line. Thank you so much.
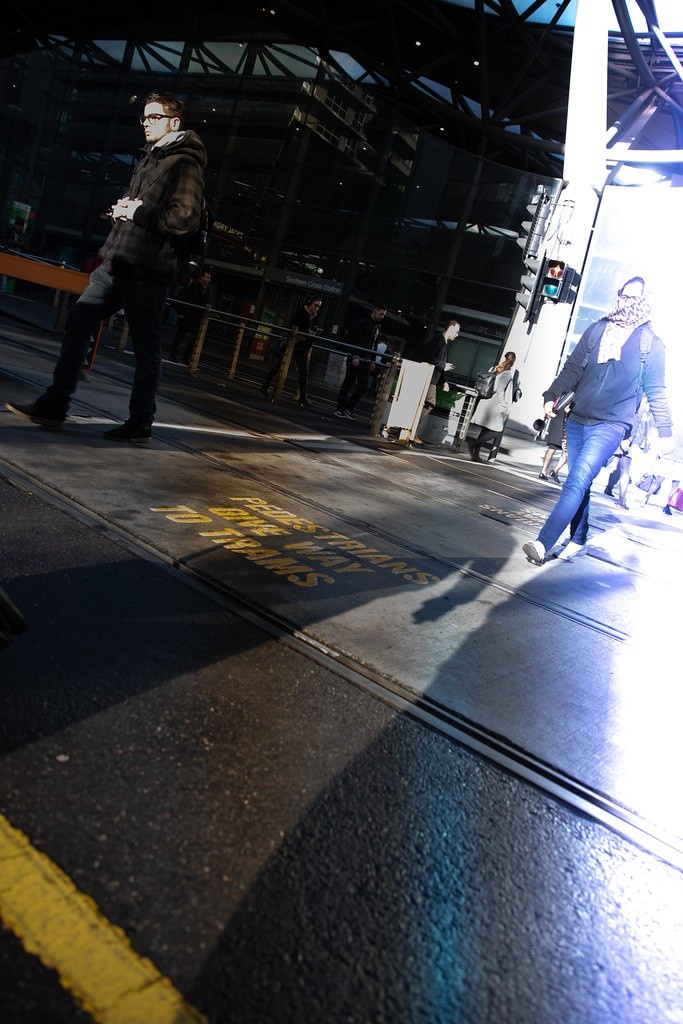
[414, 436, 425, 445]
[617, 500, 629, 510]
[471, 454, 484, 463]
[467, 441, 474, 457]
[604, 486, 614, 497]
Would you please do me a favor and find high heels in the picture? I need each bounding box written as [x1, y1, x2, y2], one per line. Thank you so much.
[299, 397, 313, 407]
[663, 505, 672, 515]
[260, 386, 270, 396]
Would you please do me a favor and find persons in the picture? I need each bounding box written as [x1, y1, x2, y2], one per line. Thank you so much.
[6, 90, 207, 444]
[522, 276, 673, 568]
[0, 215, 682, 514]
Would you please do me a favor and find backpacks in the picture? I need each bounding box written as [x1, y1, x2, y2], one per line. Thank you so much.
[474, 367, 499, 399]
[159, 191, 212, 277]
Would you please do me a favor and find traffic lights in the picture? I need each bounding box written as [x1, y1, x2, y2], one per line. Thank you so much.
[554, 269, 581, 305]
[539, 258, 569, 301]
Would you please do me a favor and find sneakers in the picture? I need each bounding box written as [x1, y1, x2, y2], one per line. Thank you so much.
[5, 399, 70, 427]
[104, 421, 154, 443]
[344, 408, 355, 420]
[552, 540, 588, 560]
[522, 541, 546, 567]
[334, 411, 347, 419]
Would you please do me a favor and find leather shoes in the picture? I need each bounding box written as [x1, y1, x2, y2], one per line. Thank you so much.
[549, 470, 560, 484]
[539, 473, 548, 480]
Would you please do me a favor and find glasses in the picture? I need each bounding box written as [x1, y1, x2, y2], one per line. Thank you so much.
[140, 112, 169, 125]
[312, 303, 321, 309]
[618, 294, 642, 302]
